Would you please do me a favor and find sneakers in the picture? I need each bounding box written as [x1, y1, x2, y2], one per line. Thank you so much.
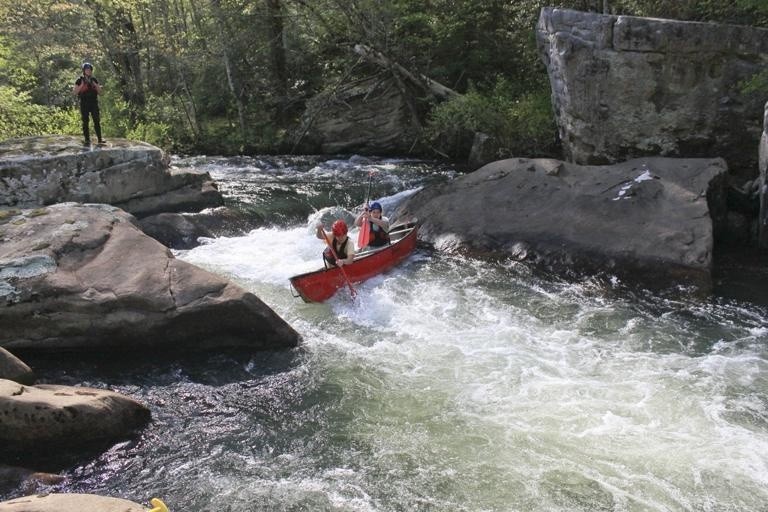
[83, 139, 107, 148]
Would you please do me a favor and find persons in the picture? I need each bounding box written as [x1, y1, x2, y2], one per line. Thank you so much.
[352, 201, 391, 251]
[74, 61, 108, 145]
[314, 218, 355, 270]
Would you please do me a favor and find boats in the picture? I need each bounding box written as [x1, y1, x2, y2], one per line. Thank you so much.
[290, 214, 423, 303]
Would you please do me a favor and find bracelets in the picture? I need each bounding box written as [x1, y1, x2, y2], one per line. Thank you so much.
[92, 84, 97, 89]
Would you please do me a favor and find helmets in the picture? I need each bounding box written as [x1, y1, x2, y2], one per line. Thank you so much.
[369, 202, 382, 212]
[332, 219, 348, 237]
[81, 62, 93, 72]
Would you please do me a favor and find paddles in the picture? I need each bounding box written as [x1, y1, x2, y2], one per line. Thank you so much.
[358, 175, 372, 247]
